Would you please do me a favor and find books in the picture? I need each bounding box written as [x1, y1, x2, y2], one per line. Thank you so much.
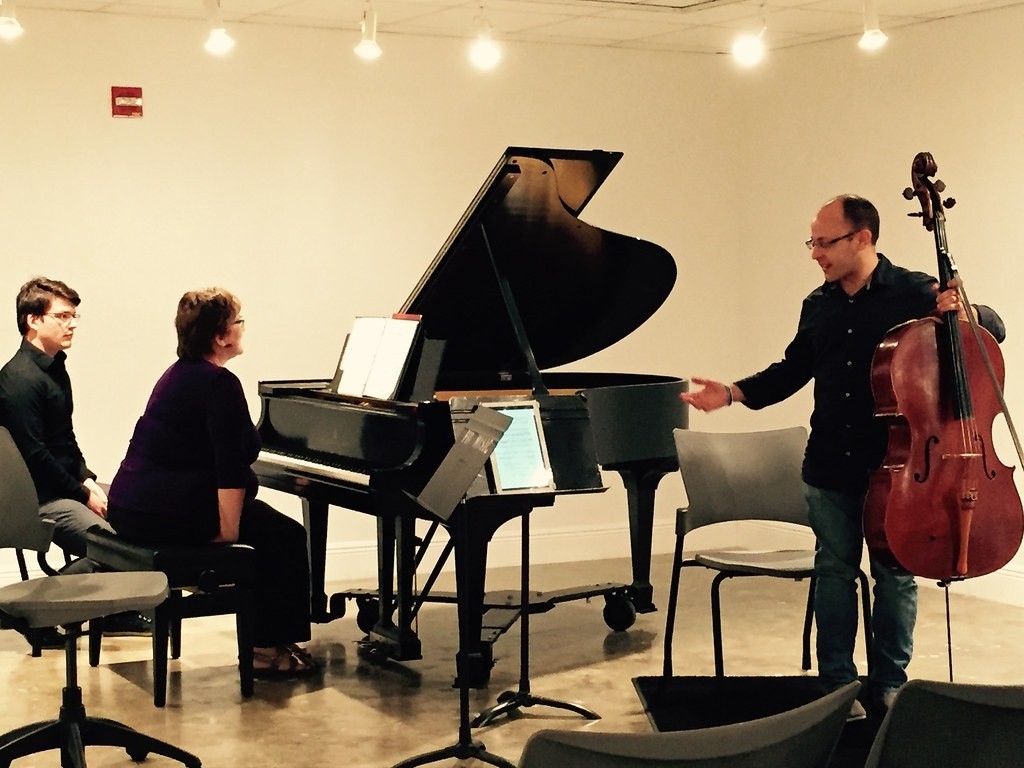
[331, 317, 420, 400]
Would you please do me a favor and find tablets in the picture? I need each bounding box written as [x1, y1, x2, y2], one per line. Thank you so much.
[479, 400, 555, 495]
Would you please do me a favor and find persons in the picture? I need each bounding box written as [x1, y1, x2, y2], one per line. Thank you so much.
[0, 277, 152, 636]
[106, 287, 321, 680]
[680, 194, 1006, 722]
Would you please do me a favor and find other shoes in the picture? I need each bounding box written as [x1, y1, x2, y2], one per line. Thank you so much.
[844, 698, 867, 725]
[883, 684, 901, 708]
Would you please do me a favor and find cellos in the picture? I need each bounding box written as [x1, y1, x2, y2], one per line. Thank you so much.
[860, 150, 1024, 683]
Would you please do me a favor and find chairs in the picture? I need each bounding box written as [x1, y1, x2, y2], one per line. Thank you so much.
[664, 426, 870, 674]
[519, 676, 1024, 767]
[0, 425, 203, 768]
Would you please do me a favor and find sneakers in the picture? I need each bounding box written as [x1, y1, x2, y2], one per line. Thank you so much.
[103, 611, 155, 636]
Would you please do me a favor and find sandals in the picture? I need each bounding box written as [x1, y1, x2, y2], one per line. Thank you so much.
[252, 644, 323, 679]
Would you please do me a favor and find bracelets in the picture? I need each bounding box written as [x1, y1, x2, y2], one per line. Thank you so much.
[725, 385, 733, 406]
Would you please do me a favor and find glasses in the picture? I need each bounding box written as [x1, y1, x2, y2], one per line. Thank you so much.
[44, 311, 81, 322]
[233, 316, 245, 326]
[805, 230, 856, 249]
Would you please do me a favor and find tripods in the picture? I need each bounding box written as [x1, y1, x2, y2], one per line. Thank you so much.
[391, 393, 602, 768]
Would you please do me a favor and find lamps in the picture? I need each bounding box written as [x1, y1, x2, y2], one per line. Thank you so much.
[353, 9, 382, 60]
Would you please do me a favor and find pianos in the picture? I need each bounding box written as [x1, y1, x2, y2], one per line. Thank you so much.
[248, 146, 692, 689]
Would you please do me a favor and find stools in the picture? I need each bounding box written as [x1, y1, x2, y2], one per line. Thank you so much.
[83, 524, 254, 707]
